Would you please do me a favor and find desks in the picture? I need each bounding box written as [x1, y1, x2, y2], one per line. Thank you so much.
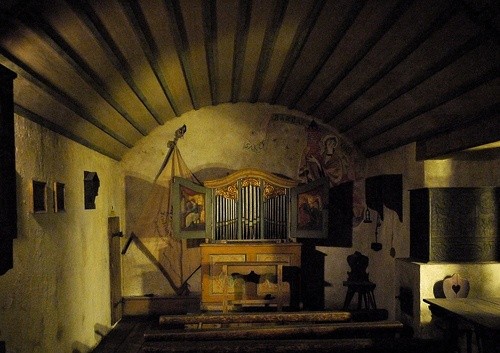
[422, 293, 500, 353]
[199, 241, 302, 309]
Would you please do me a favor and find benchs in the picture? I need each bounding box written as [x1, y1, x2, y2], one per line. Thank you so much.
[139, 305, 441, 353]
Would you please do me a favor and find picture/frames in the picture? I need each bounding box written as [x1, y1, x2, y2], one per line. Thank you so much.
[52, 180, 67, 214]
[28, 177, 49, 215]
